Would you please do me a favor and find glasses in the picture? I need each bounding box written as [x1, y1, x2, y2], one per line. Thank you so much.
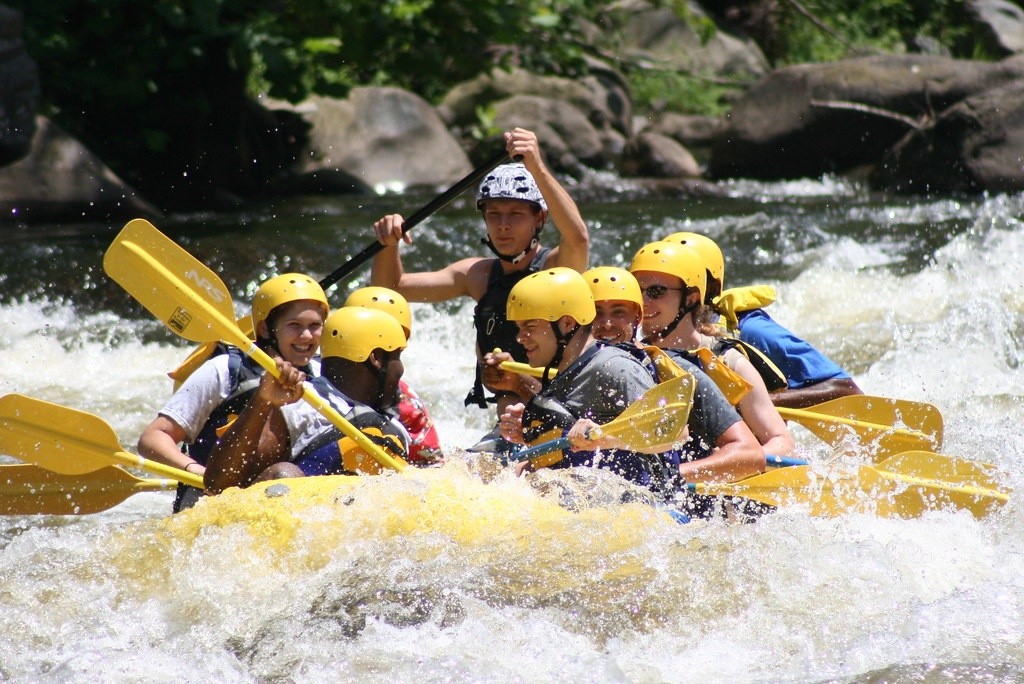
[641, 285, 684, 298]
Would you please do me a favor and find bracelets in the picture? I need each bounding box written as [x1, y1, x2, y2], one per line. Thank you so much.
[184, 461, 198, 471]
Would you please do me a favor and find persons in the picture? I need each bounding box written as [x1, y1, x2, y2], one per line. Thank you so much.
[137, 273, 442, 515]
[480, 232, 867, 527]
[371, 126, 590, 460]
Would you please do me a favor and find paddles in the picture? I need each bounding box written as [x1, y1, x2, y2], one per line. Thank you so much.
[686, 463, 888, 518]
[103, 218, 401, 474]
[172, 148, 524, 395]
[764, 449, 1013, 518]
[0, 392, 206, 490]
[0, 464, 180, 515]
[496, 373, 696, 460]
[775, 393, 944, 463]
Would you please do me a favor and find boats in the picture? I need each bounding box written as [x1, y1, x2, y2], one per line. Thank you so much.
[0, 471, 681, 652]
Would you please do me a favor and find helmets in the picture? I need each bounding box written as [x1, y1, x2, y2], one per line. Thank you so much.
[580, 266, 644, 325]
[319, 305, 408, 363]
[475, 163, 548, 212]
[629, 240, 708, 306]
[345, 286, 412, 340]
[252, 272, 330, 333]
[506, 266, 597, 325]
[663, 231, 724, 295]
